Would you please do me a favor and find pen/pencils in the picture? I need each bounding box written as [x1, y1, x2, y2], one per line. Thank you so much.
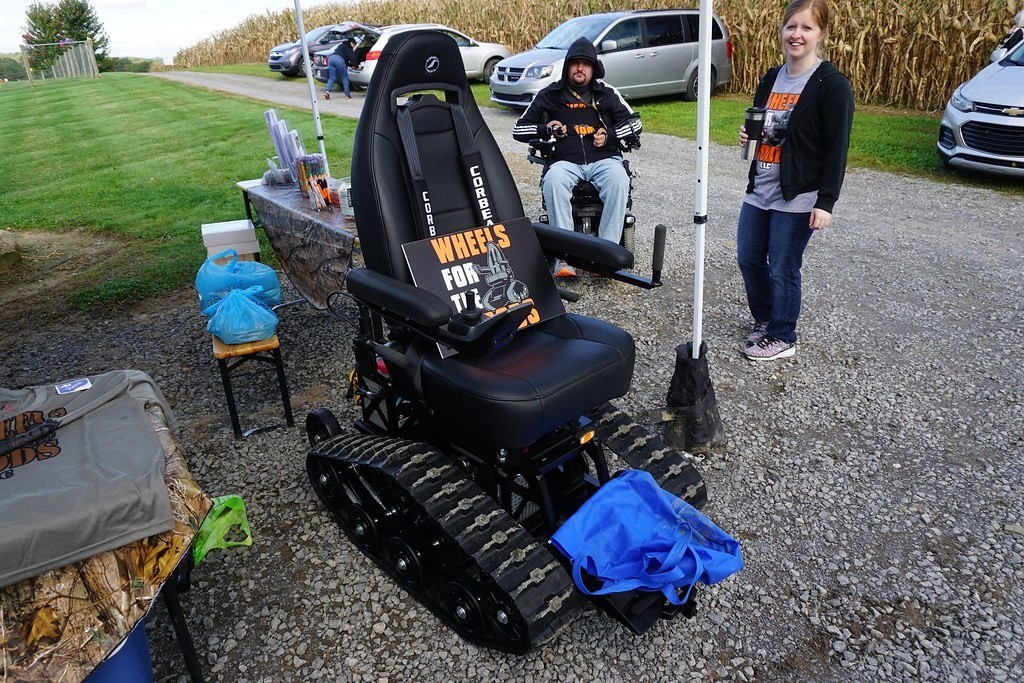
[295, 155, 332, 209]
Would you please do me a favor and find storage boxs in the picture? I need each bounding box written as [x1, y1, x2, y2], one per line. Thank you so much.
[80, 618, 153, 683]
[200, 219, 261, 258]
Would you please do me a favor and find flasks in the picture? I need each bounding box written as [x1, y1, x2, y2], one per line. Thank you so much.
[740, 107, 767, 160]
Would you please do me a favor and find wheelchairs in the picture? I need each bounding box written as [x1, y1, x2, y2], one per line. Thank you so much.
[304, 28, 712, 655]
[524, 119, 636, 276]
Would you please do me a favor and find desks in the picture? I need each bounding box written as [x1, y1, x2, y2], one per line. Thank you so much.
[0, 369, 214, 683]
[235, 178, 383, 343]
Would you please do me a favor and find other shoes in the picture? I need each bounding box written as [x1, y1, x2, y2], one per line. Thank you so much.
[324, 92, 330, 100]
[345, 96, 352, 99]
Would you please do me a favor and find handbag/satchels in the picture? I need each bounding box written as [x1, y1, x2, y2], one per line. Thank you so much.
[202, 285, 279, 345]
[195, 247, 281, 308]
[548, 468, 743, 606]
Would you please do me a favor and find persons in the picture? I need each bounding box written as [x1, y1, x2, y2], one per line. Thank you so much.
[512, 36, 642, 278]
[325, 37, 360, 101]
[736, 0, 855, 361]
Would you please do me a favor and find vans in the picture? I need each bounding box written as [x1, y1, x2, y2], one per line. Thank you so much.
[488, 7, 735, 115]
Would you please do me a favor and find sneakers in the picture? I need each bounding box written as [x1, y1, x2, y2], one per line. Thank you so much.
[742, 330, 795, 360]
[747, 322, 769, 345]
[553, 258, 576, 277]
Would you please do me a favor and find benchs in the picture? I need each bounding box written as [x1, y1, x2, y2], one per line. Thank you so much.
[207, 253, 295, 440]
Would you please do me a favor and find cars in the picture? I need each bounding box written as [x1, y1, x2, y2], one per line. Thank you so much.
[267, 22, 383, 85]
[333, 23, 517, 92]
[934, 37, 1024, 177]
